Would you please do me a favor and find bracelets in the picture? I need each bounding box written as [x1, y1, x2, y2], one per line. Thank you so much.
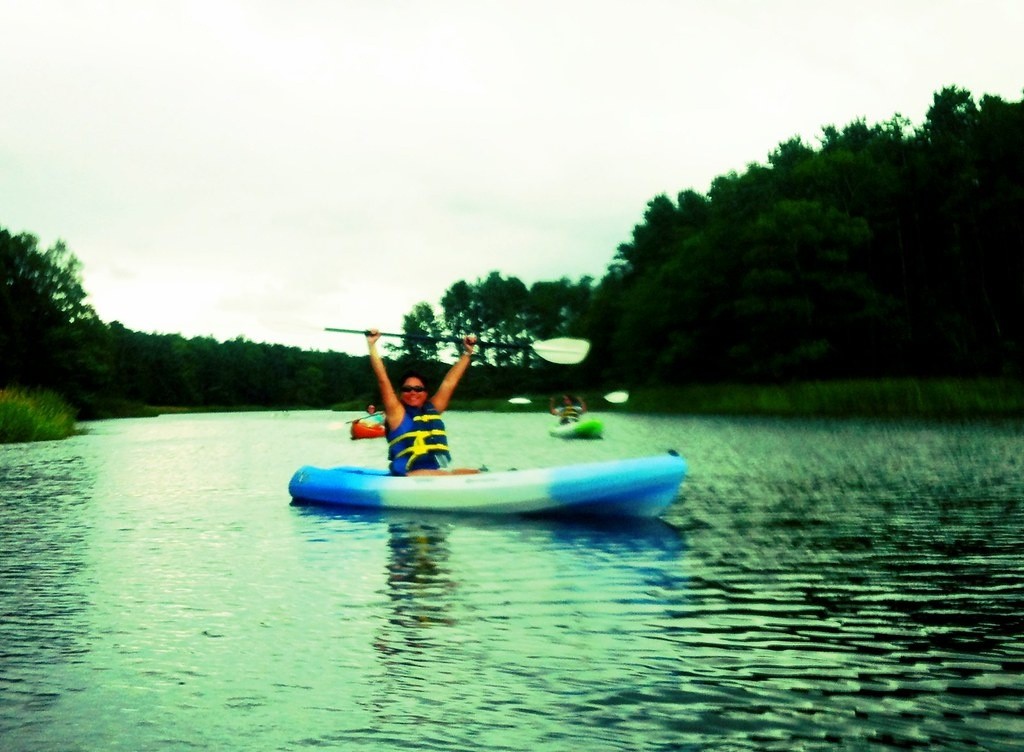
[463, 351, 471, 356]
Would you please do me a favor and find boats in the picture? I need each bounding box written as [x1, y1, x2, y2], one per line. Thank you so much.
[549, 419, 604, 438]
[351, 418, 386, 438]
[288, 448, 687, 518]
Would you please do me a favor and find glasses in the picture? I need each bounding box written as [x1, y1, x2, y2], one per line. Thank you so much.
[400, 385, 427, 392]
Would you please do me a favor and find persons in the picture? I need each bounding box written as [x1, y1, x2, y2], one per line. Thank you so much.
[364, 330, 481, 476]
[354, 404, 386, 431]
[549, 394, 587, 426]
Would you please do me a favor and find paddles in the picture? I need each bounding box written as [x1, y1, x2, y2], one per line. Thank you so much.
[326, 411, 384, 432]
[508, 389, 630, 405]
[324, 324, 595, 366]
